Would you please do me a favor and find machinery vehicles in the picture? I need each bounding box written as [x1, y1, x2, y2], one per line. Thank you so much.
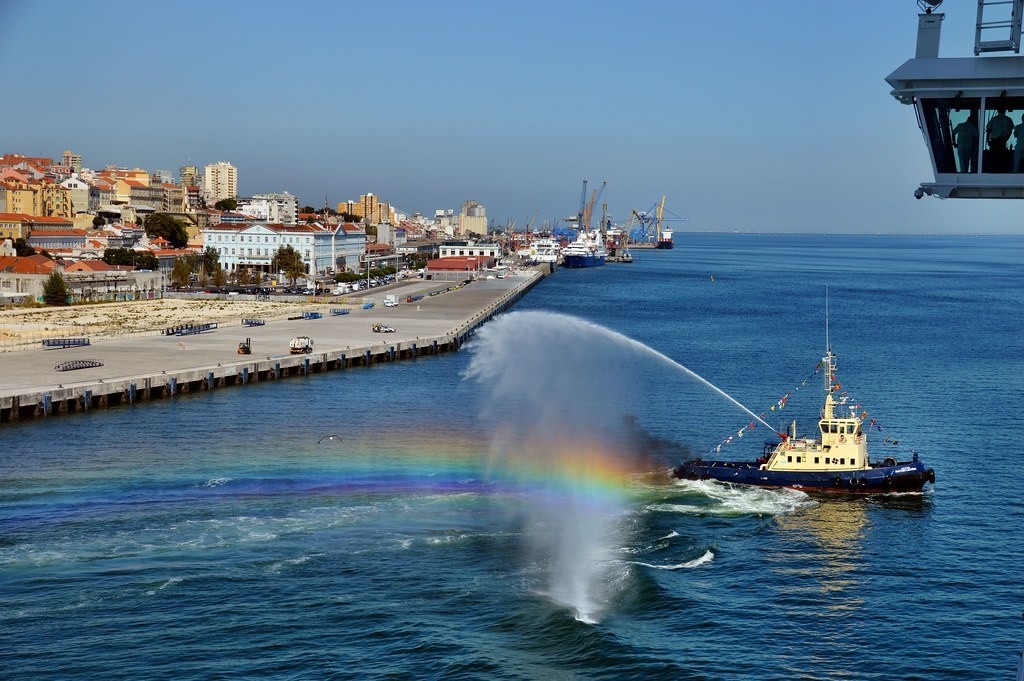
[239, 337, 251, 354]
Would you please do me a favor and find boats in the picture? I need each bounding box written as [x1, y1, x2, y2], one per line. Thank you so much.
[527, 228, 623, 268]
[671, 284, 935, 502]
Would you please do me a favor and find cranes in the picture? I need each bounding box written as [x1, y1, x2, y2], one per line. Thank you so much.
[576, 176, 688, 250]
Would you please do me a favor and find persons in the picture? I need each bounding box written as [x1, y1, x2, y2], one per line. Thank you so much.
[952, 110, 1024, 173]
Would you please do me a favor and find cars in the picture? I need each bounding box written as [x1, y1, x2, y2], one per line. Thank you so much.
[373, 323, 396, 334]
[204, 266, 429, 308]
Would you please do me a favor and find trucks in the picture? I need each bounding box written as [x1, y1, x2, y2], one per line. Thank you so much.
[290, 336, 315, 355]
